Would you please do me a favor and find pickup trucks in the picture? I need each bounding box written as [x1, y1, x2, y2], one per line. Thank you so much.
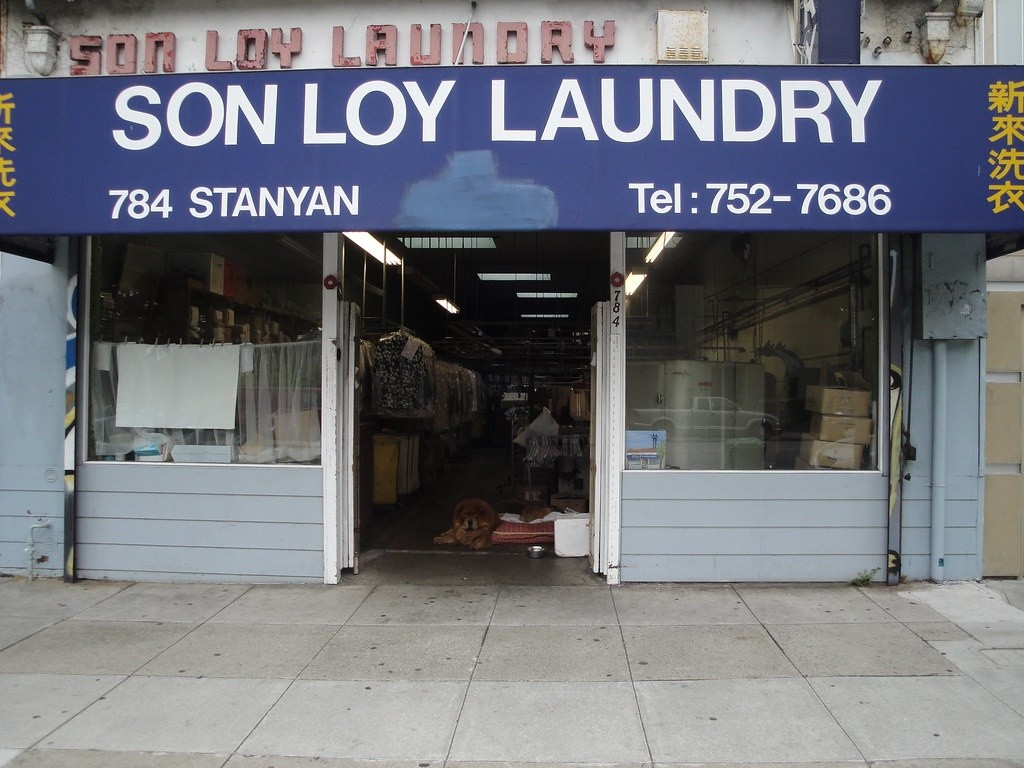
[631, 396, 783, 441]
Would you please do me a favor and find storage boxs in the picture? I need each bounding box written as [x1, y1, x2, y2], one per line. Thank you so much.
[793, 382, 873, 470]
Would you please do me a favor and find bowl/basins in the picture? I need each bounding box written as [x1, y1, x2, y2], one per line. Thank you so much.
[526, 545, 545, 559]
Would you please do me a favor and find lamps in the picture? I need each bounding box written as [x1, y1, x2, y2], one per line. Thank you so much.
[622, 270, 649, 298]
[433, 293, 461, 315]
[342, 230, 403, 268]
[642, 228, 677, 264]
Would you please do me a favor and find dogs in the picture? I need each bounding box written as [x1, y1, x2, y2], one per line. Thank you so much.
[519, 505, 565, 522]
[433, 498, 502, 551]
